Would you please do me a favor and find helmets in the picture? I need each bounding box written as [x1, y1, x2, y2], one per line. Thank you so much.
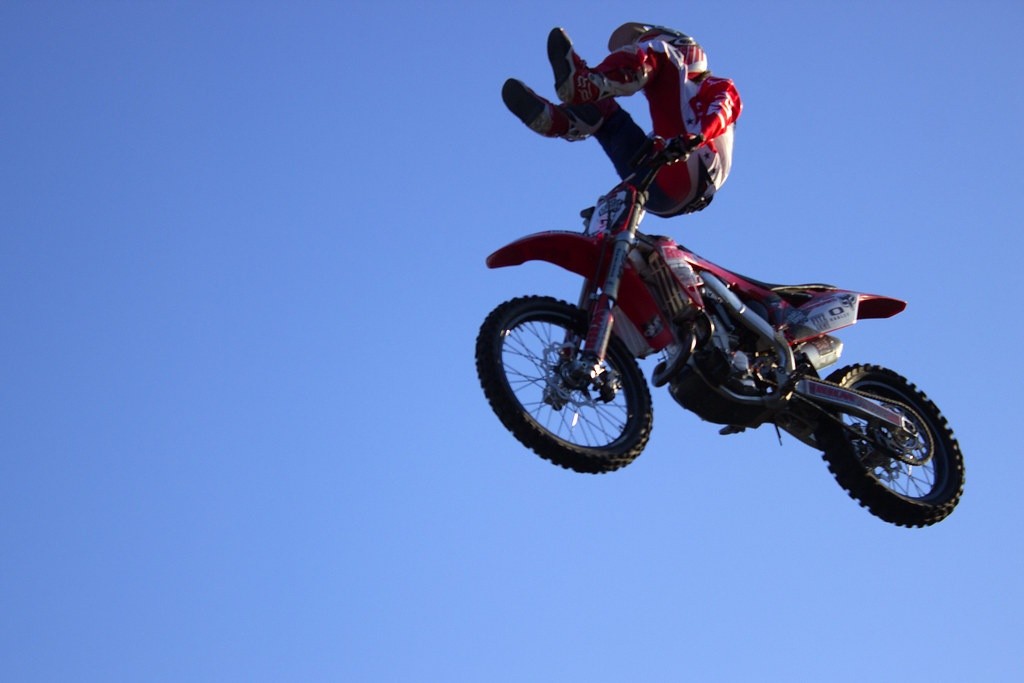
[607, 19, 708, 81]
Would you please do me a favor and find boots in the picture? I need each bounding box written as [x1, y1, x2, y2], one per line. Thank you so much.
[547, 26, 649, 106]
[502, 78, 603, 143]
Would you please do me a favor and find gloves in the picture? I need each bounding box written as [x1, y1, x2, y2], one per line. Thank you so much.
[650, 131, 702, 167]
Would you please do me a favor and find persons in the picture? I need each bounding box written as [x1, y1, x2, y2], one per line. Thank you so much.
[502, 22, 742, 218]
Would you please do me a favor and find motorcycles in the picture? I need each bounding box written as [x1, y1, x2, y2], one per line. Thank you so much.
[471, 129, 967, 529]
